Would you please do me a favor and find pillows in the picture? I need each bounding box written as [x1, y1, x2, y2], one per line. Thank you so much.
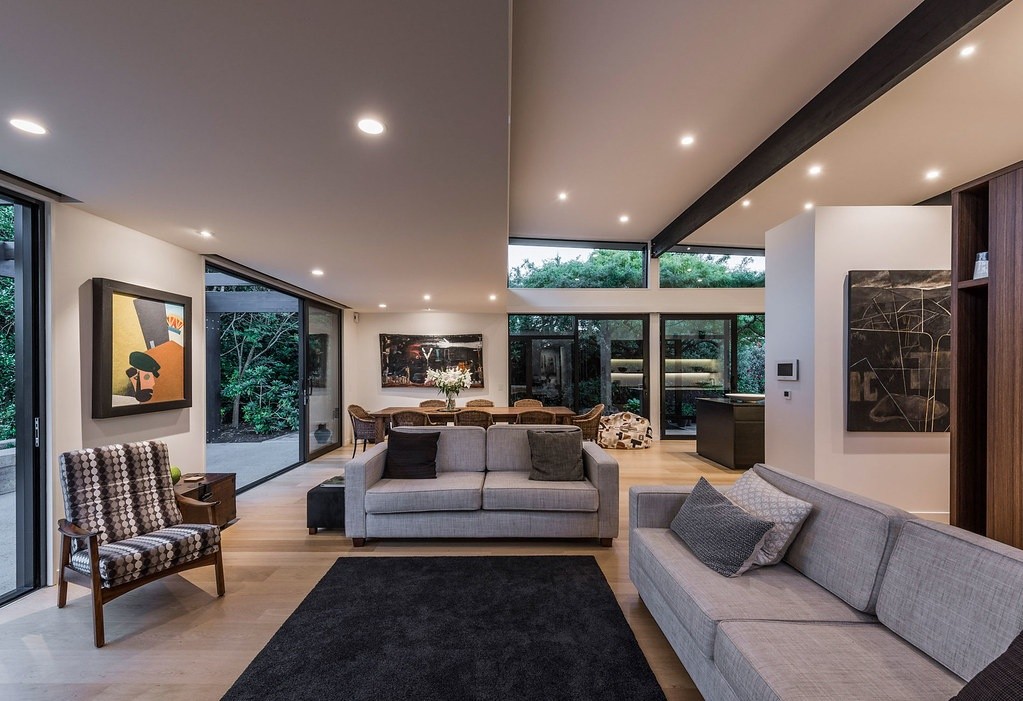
[526, 428, 583, 480]
[947, 631, 1023, 701]
[727, 468, 813, 574]
[670, 477, 774, 575]
[381, 430, 442, 481]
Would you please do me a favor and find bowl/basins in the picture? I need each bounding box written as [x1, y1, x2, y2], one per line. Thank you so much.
[617, 366, 627, 373]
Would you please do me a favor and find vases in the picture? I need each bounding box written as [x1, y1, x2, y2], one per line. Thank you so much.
[446, 384, 458, 410]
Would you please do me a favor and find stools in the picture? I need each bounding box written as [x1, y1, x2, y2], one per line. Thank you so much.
[306, 476, 346, 535]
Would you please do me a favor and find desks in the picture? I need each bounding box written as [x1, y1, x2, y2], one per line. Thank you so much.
[370, 405, 576, 443]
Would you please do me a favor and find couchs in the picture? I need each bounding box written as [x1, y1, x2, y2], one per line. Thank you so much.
[346, 425, 617, 549]
[629, 462, 1023, 701]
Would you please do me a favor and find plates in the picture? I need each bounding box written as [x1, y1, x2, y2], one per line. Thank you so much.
[725, 393, 765, 401]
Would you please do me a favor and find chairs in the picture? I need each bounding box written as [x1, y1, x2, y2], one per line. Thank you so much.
[58, 440, 225, 647]
[514, 400, 543, 408]
[515, 410, 556, 425]
[573, 403, 604, 444]
[467, 399, 494, 406]
[453, 409, 495, 427]
[392, 410, 439, 430]
[420, 400, 447, 426]
[347, 405, 376, 458]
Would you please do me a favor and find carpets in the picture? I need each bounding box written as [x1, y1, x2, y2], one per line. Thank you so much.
[218, 556, 668, 701]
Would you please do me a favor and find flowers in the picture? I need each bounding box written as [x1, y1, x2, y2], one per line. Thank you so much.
[424, 366, 472, 394]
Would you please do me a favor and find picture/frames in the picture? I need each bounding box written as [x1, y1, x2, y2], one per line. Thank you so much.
[89, 277, 193, 420]
[379, 333, 485, 388]
[307, 333, 330, 388]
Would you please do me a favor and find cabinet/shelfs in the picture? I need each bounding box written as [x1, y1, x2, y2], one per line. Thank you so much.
[696, 399, 765, 472]
[171, 471, 240, 530]
[948, 166, 1023, 550]
